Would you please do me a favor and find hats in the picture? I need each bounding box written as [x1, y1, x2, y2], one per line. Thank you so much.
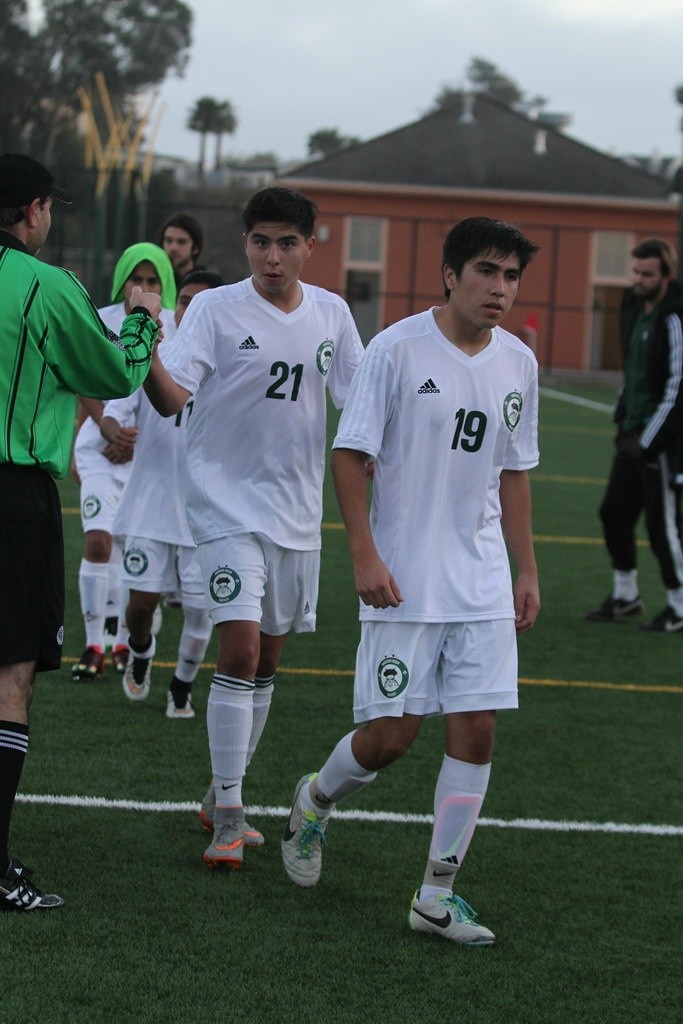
[0, 152, 73, 208]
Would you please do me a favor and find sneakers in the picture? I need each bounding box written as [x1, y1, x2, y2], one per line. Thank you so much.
[165, 687, 195, 718]
[111, 647, 130, 672]
[408, 888, 496, 946]
[204, 820, 247, 871]
[70, 646, 106, 683]
[585, 595, 644, 622]
[199, 798, 264, 847]
[121, 652, 153, 702]
[0, 858, 63, 911]
[651, 603, 683, 631]
[281, 772, 331, 888]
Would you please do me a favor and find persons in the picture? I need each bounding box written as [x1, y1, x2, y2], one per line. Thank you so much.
[100, 272, 227, 720]
[0, 150, 164, 912]
[69, 242, 177, 680]
[139, 185, 375, 870]
[280, 216, 542, 944]
[583, 236, 683, 633]
[159, 213, 227, 289]
